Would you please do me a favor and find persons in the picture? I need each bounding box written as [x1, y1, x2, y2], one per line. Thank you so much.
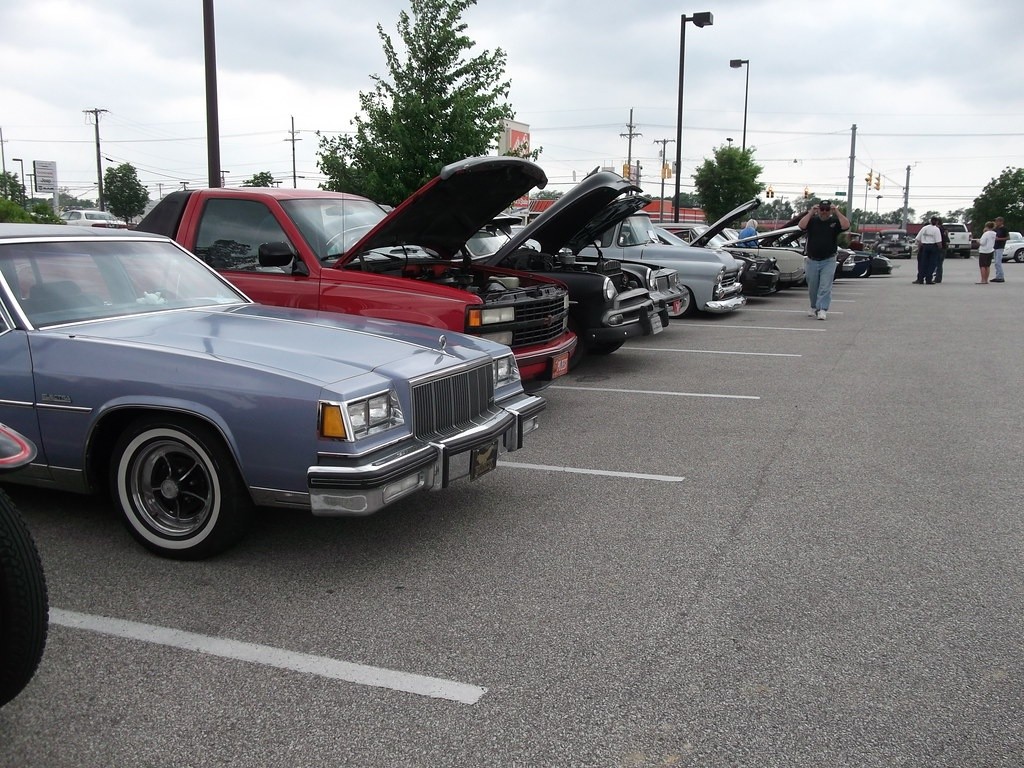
[976, 216, 1010, 283]
[911, 216, 950, 285]
[798, 199, 851, 319]
[737, 219, 759, 248]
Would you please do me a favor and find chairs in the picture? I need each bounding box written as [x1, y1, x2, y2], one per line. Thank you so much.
[29, 280, 79, 312]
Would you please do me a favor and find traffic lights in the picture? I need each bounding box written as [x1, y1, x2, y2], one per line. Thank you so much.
[875, 177, 880, 191]
[867, 173, 872, 186]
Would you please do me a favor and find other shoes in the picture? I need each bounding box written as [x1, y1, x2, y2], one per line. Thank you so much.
[807, 308, 817, 317]
[933, 279, 941, 283]
[912, 280, 924, 284]
[926, 281, 935, 284]
[817, 310, 826, 320]
[990, 278, 1004, 282]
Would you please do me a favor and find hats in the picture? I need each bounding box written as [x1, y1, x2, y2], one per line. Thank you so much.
[820, 200, 831, 207]
[995, 217, 1005, 221]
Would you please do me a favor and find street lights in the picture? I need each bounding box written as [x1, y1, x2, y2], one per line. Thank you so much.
[675, 12, 714, 222]
[730, 59, 749, 152]
[12, 158, 25, 193]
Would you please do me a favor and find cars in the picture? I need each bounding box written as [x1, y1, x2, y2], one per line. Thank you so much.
[872, 228, 912, 259]
[0, 222, 546, 562]
[652, 197, 893, 296]
[333, 168, 669, 369]
[27, 156, 579, 394]
[60, 211, 127, 231]
[1003, 232, 1024, 262]
[512, 208, 749, 319]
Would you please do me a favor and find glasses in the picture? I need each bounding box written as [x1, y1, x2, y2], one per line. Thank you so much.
[820, 208, 830, 212]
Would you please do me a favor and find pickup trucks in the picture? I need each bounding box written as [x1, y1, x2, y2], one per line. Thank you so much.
[942, 222, 973, 258]
